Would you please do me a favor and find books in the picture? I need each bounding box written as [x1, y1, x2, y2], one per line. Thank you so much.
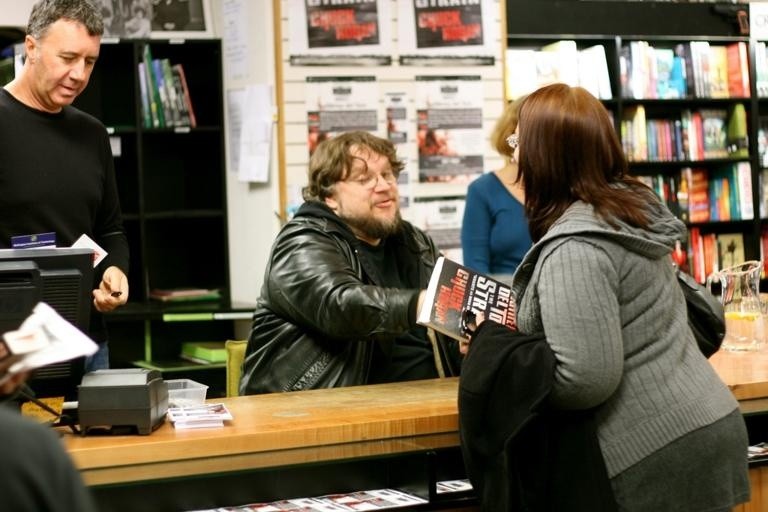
[756, 42, 768, 98]
[167, 402, 233, 422]
[621, 103, 748, 162]
[154, 286, 220, 301]
[1, 299, 104, 383]
[760, 227, 768, 279]
[671, 227, 744, 283]
[173, 421, 224, 430]
[416, 255, 517, 347]
[136, 41, 198, 128]
[504, 40, 612, 100]
[636, 162, 754, 223]
[759, 168, 768, 220]
[621, 42, 751, 99]
[757, 115, 768, 169]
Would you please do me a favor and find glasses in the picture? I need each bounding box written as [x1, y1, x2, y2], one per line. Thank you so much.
[344, 170, 393, 187]
[504, 132, 521, 149]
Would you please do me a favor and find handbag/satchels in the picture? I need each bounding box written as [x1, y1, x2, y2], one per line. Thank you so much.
[676, 266, 726, 359]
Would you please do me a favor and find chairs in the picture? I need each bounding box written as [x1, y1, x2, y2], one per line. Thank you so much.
[426, 451, 482, 512]
[225, 340, 246, 398]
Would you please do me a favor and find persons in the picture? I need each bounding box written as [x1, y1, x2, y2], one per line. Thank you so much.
[237, 131, 462, 399]
[1, 328, 98, 511]
[460, 94, 533, 289]
[0, 1, 129, 372]
[458, 83, 751, 511]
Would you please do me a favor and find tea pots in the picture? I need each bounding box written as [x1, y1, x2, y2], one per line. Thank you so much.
[704, 260, 766, 353]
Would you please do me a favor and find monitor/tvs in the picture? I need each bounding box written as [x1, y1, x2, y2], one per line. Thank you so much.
[0, 247, 95, 401]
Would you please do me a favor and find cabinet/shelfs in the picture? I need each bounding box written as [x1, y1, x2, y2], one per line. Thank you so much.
[0, 37, 236, 399]
[507, 32, 768, 295]
